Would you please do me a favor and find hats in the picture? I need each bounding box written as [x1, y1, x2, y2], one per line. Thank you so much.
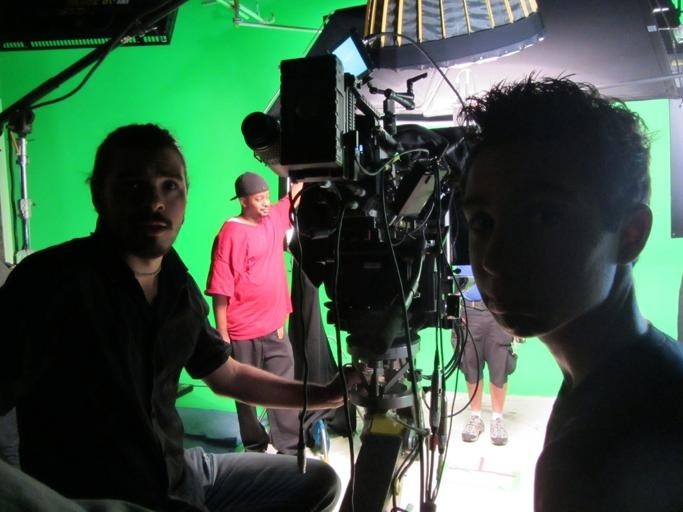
[230, 173, 268, 201]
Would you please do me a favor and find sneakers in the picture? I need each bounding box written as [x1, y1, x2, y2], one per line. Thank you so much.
[490, 418, 509, 445]
[462, 414, 485, 441]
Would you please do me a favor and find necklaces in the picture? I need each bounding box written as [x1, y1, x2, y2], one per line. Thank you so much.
[133, 265, 161, 275]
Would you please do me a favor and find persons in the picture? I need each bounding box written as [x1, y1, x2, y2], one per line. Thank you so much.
[449, 209, 525, 445]
[0, 125, 386, 512]
[205, 173, 304, 455]
[458, 72, 682, 511]
